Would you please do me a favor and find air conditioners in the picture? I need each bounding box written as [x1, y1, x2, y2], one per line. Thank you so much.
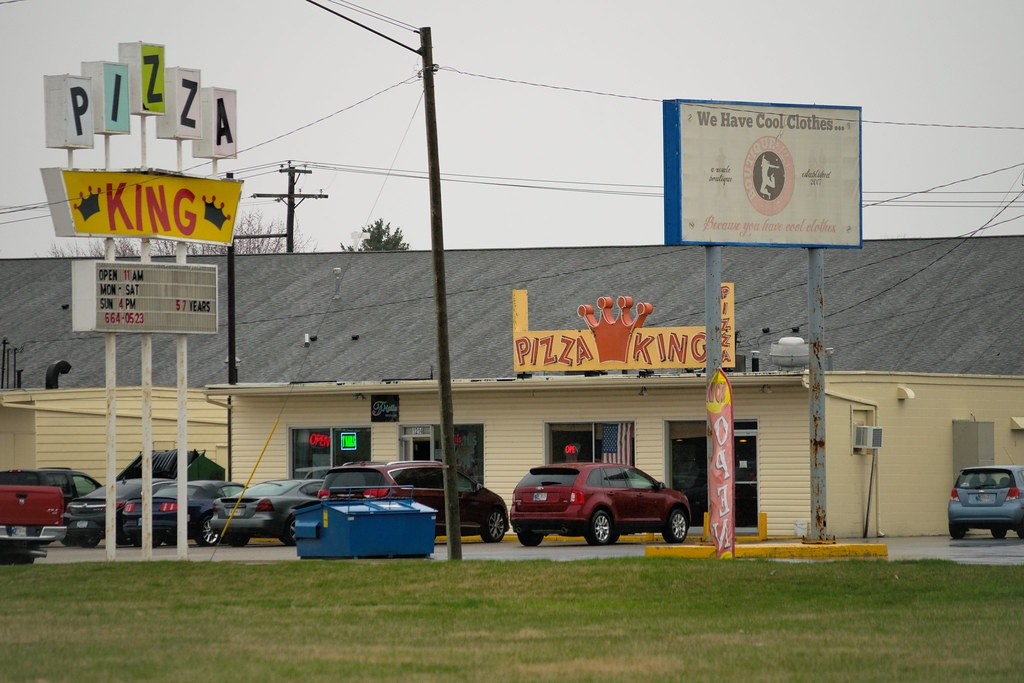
[854, 425, 883, 449]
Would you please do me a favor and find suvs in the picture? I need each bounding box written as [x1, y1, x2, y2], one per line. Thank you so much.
[294, 465, 336, 479]
[0, 467, 104, 547]
[319, 460, 509, 544]
[510, 462, 692, 545]
[948, 465, 1024, 539]
[63, 478, 177, 548]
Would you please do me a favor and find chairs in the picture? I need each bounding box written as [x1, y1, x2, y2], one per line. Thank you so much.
[969, 477, 980, 486]
[984, 479, 996, 485]
[999, 477, 1010, 485]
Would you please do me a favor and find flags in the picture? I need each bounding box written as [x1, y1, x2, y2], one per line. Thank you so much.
[601, 423, 633, 466]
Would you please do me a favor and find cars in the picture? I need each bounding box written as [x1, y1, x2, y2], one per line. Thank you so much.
[211, 480, 325, 548]
[122, 481, 249, 547]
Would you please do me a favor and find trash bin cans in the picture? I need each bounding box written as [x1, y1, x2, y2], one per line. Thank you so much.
[115, 449, 225, 482]
[294, 499, 439, 559]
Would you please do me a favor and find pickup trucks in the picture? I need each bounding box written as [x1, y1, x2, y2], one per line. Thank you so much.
[0, 485, 68, 564]
